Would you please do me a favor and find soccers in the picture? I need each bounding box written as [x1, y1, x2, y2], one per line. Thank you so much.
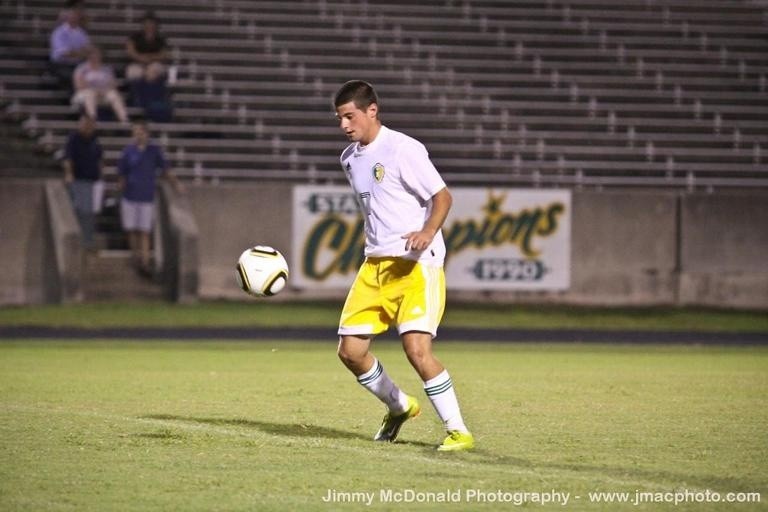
[235, 244, 289, 298]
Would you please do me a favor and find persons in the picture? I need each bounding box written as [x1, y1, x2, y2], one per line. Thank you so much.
[122, 12, 175, 107]
[330, 79, 477, 454]
[113, 118, 186, 273]
[61, 111, 105, 258]
[48, 6, 94, 80]
[71, 45, 129, 122]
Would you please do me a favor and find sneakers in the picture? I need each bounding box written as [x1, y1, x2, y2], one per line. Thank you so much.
[433, 430, 474, 451]
[374, 395, 421, 442]
